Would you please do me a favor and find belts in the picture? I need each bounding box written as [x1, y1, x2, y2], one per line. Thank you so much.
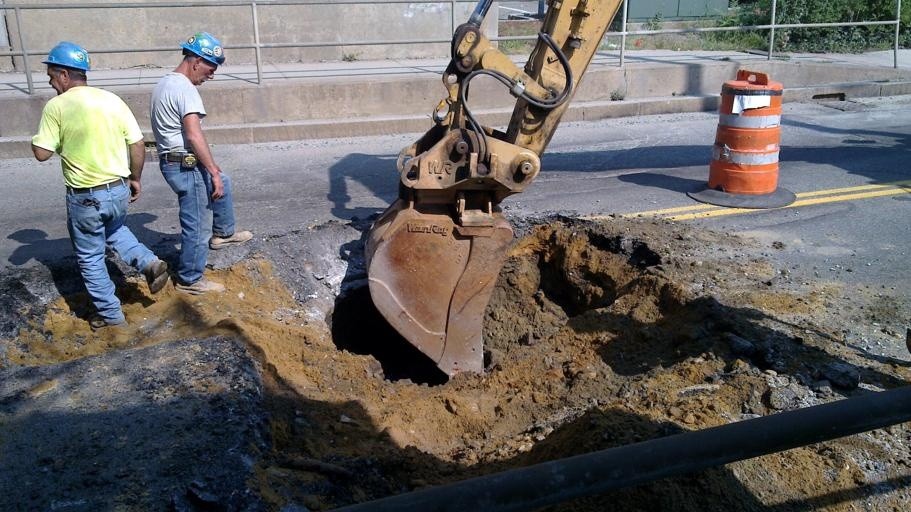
[66, 177, 127, 194]
[161, 154, 183, 162]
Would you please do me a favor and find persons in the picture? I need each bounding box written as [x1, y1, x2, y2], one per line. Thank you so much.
[149, 28, 255, 296]
[30, 41, 171, 327]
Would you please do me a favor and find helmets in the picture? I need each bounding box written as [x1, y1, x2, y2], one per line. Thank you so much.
[179, 30, 225, 67]
[41, 41, 92, 71]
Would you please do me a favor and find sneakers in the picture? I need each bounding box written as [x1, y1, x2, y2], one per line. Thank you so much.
[173, 278, 226, 297]
[209, 230, 253, 249]
[89, 315, 128, 331]
[143, 258, 168, 294]
